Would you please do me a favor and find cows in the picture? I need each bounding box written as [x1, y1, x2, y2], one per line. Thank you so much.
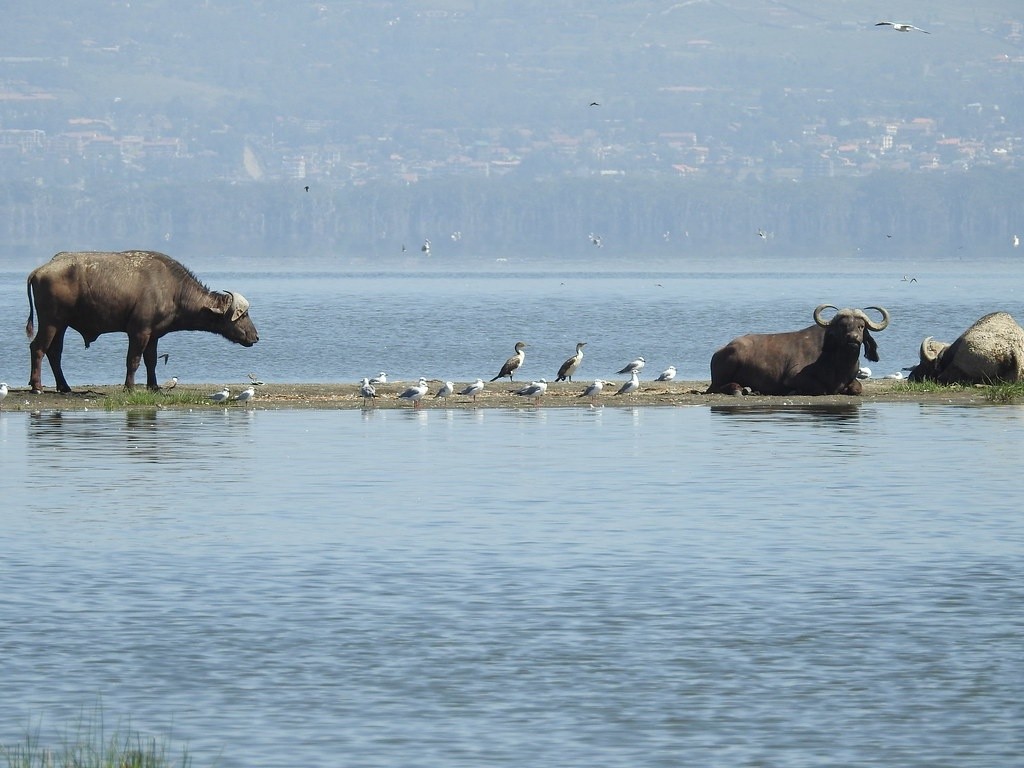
[899, 310, 1024, 385]
[686, 302, 891, 398]
[25, 249, 260, 396]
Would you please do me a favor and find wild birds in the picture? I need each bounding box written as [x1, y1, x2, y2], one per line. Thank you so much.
[247, 373, 266, 385]
[586, 230, 604, 249]
[900, 275, 918, 284]
[874, 20, 931, 35]
[653, 283, 665, 288]
[856, 366, 872, 379]
[401, 243, 407, 252]
[160, 375, 183, 392]
[203, 385, 258, 407]
[755, 229, 769, 239]
[1012, 234, 1020, 248]
[589, 102, 601, 107]
[303, 185, 311, 193]
[886, 234, 892, 238]
[882, 371, 903, 380]
[420, 238, 436, 257]
[158, 353, 170, 365]
[0, 382, 12, 401]
[357, 340, 680, 409]
